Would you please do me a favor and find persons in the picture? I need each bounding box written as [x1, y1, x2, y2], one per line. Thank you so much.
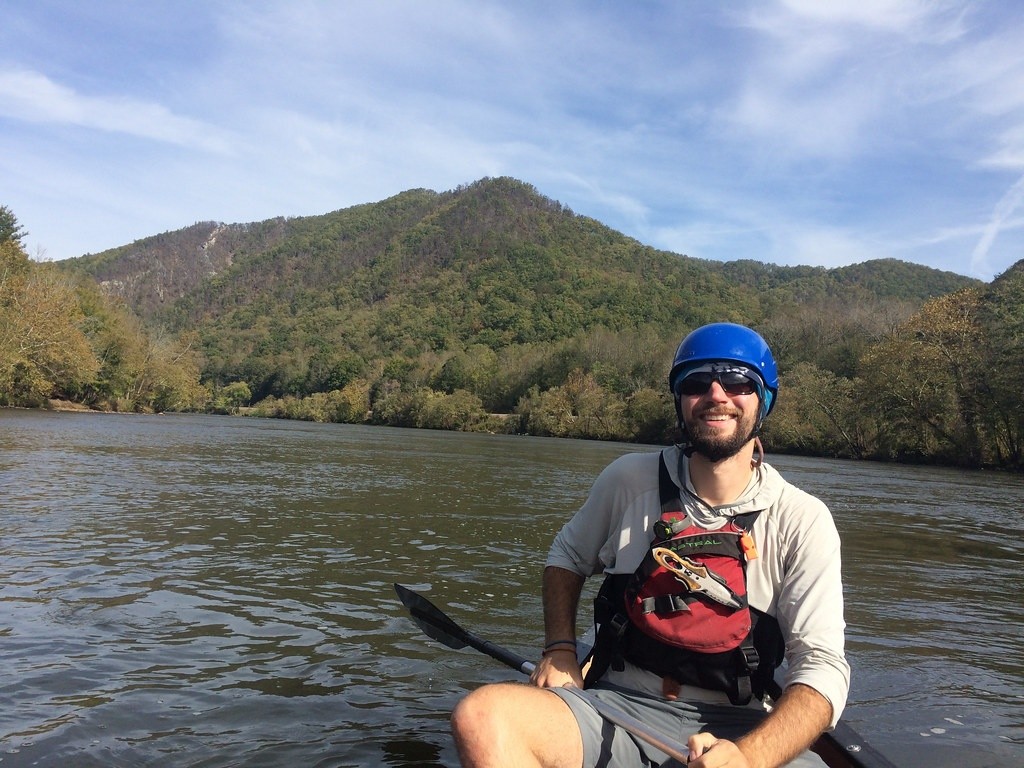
[451, 322, 852, 768]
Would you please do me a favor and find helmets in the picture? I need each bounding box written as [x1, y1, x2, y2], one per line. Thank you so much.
[668, 322, 779, 417]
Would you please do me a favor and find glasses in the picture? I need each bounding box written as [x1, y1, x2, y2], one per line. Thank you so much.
[675, 371, 758, 396]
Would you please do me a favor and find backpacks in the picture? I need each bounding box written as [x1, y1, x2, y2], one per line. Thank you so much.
[583, 449, 789, 706]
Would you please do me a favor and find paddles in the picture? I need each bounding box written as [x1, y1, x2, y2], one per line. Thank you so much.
[390, 579, 702, 767]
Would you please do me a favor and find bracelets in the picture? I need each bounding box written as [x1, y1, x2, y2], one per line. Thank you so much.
[543, 641, 578, 658]
